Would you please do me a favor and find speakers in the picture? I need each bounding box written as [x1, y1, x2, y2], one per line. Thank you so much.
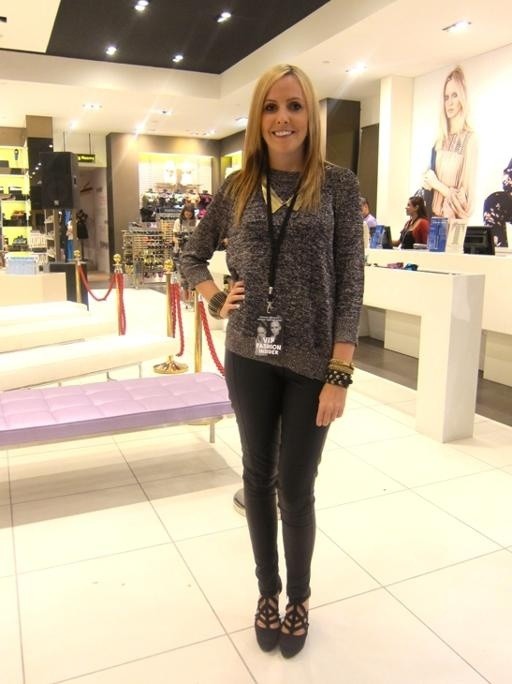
[39, 152, 79, 209]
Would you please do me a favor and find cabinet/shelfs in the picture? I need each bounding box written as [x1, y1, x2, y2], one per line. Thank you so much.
[121, 216, 197, 284]
[0, 166, 29, 250]
[44, 208, 65, 273]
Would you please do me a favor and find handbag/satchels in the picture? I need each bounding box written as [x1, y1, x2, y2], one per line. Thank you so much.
[413, 188, 432, 215]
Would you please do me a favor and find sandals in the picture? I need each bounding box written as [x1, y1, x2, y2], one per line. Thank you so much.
[254, 586, 311, 658]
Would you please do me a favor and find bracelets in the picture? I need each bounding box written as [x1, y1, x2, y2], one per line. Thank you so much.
[208, 292, 227, 320]
[325, 359, 355, 388]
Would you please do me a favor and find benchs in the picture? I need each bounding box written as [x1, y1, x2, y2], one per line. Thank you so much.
[0, 301, 235, 446]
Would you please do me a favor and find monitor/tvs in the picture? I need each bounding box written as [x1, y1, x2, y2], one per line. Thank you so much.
[463, 225, 495, 255]
[370, 226, 393, 249]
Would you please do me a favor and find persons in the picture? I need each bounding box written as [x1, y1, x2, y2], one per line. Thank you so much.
[422, 66, 478, 220]
[363, 195, 430, 249]
[174, 204, 202, 262]
[180, 64, 364, 658]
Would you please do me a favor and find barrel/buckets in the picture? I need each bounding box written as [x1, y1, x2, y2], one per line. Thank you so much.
[427, 217, 448, 252]
[445, 218, 467, 253]
